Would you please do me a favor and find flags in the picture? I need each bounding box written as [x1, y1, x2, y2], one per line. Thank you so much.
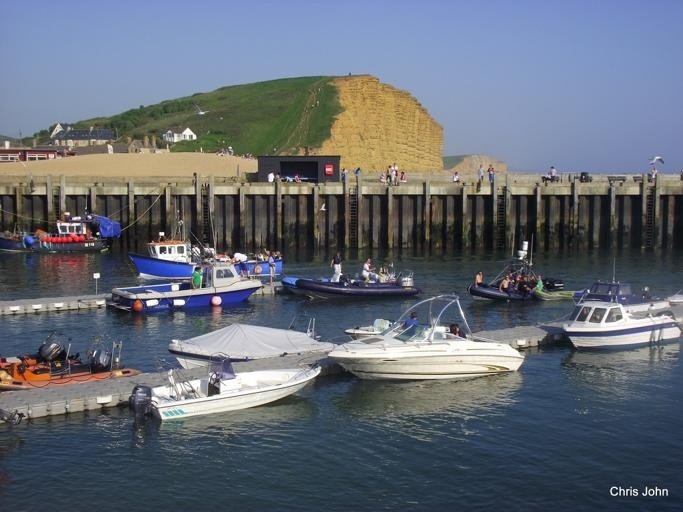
[48, 122, 64, 140]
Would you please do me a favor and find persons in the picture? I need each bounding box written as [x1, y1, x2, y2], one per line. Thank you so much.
[267, 251, 276, 276]
[361, 257, 376, 287]
[190, 244, 201, 263]
[546, 171, 551, 181]
[190, 266, 201, 289]
[214, 144, 255, 161]
[477, 164, 485, 186]
[474, 271, 483, 288]
[267, 171, 274, 182]
[497, 270, 543, 300]
[379, 163, 406, 187]
[284, 176, 293, 182]
[226, 252, 250, 279]
[651, 167, 658, 184]
[454, 323, 467, 339]
[452, 171, 461, 186]
[274, 171, 279, 180]
[378, 262, 390, 285]
[549, 166, 557, 184]
[486, 164, 495, 184]
[447, 324, 458, 339]
[293, 174, 301, 183]
[329, 250, 342, 283]
[194, 244, 201, 262]
[402, 312, 420, 336]
[354, 167, 360, 176]
[341, 167, 347, 180]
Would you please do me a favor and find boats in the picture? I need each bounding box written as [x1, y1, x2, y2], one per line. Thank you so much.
[326, 294, 525, 381]
[282, 277, 422, 300]
[344, 318, 450, 340]
[0, 330, 145, 389]
[148, 366, 322, 421]
[105, 261, 262, 313]
[126, 209, 284, 283]
[0, 211, 121, 253]
[467, 233, 682, 351]
[168, 317, 338, 369]
